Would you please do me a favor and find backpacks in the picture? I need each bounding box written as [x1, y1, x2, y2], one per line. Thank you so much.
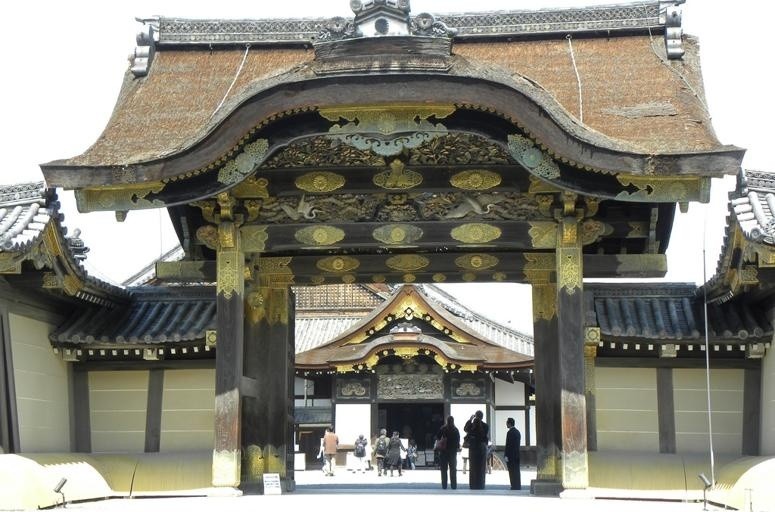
[354, 444, 364, 457]
[377, 437, 387, 455]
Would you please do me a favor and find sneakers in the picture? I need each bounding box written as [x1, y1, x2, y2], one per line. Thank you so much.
[325, 473, 333, 476]
[378, 467, 402, 477]
[353, 471, 365, 474]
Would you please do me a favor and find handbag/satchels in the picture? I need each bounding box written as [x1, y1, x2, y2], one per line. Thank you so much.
[463, 436, 471, 447]
[437, 439, 447, 450]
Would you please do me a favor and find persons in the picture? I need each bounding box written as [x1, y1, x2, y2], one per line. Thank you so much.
[504, 418, 521, 490]
[320, 426, 418, 476]
[437, 417, 460, 489]
[464, 410, 488, 489]
[461, 436, 470, 474]
[486, 437, 492, 474]
